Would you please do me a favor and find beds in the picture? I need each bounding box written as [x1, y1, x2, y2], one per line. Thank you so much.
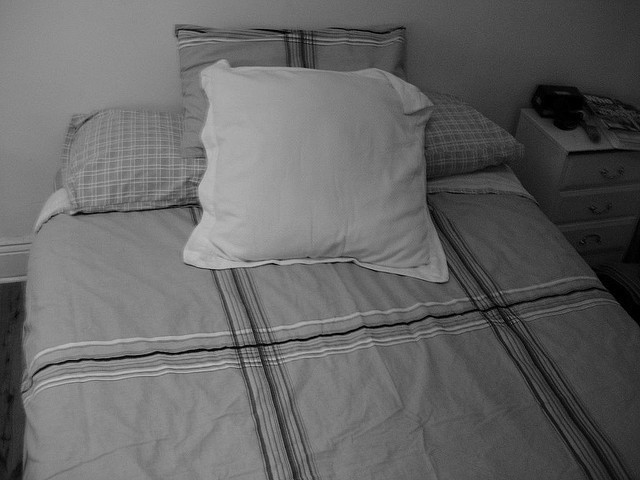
[23, 143, 640, 479]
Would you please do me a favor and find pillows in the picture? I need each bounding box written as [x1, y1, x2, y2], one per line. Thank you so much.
[416, 91, 525, 178]
[174, 22, 404, 158]
[61, 89, 205, 216]
[181, 57, 451, 285]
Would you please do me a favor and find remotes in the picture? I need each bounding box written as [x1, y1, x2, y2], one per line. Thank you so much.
[584, 125, 602, 142]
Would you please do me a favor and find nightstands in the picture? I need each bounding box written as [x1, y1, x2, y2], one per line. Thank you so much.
[516, 107, 639, 256]
